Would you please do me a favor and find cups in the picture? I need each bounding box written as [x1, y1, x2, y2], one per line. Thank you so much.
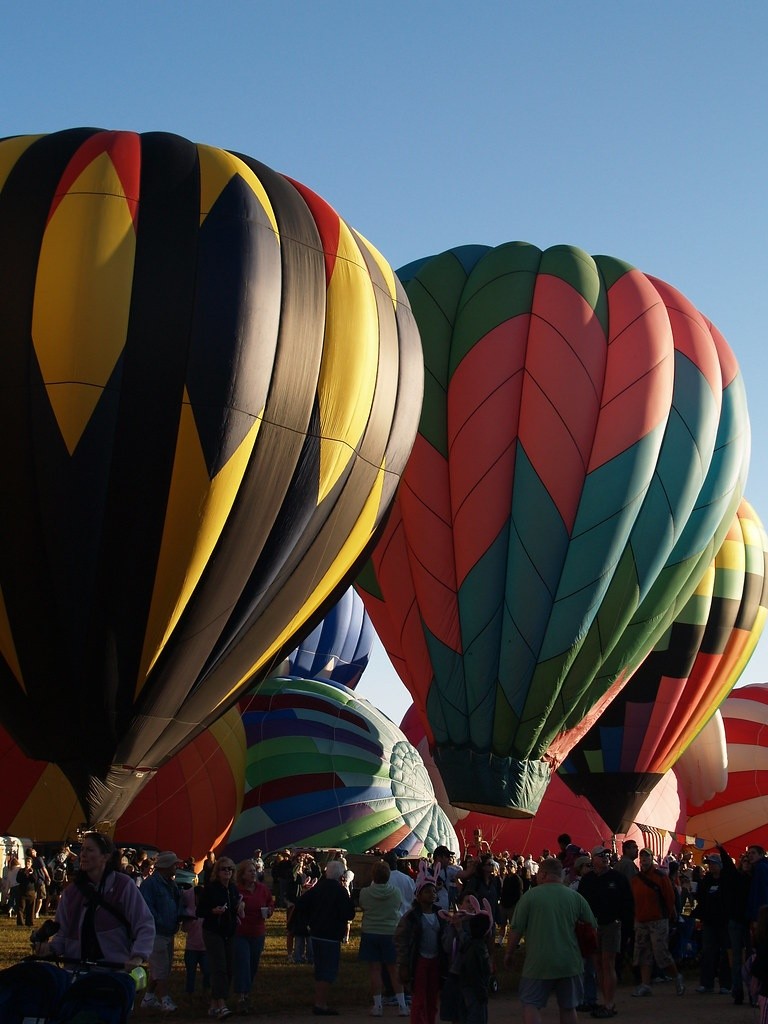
[261, 907, 269, 918]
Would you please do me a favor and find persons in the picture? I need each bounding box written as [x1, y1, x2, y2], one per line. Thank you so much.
[139, 851, 183, 1014]
[250, 848, 355, 1016]
[358, 834, 639, 1024]
[118, 848, 156, 890]
[194, 856, 273, 1019]
[630, 838, 768, 1024]
[181, 885, 211, 1005]
[30, 833, 156, 1017]
[186, 851, 217, 884]
[0, 844, 79, 929]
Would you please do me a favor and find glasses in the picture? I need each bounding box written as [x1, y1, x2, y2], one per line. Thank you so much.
[485, 862, 495, 866]
[218, 866, 236, 871]
[582, 863, 591, 868]
[596, 853, 610, 858]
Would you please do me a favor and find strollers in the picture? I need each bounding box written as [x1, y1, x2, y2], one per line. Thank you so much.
[0, 952, 148, 1024]
[410, 918, 506, 994]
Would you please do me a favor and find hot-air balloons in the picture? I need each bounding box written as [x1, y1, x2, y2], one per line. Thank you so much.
[265, 586, 377, 693]
[352, 238, 751, 856]
[549, 493, 768, 860]
[0, 125, 427, 847]
[396, 698, 684, 865]
[0, 704, 250, 880]
[220, 674, 462, 869]
[679, 680, 768, 861]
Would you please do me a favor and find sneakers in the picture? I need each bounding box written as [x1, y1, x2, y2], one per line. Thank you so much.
[140, 988, 417, 1020]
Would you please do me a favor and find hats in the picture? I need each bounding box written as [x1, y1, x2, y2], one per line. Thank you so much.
[591, 846, 611, 858]
[703, 854, 723, 868]
[573, 856, 592, 869]
[155, 851, 185, 869]
[640, 847, 654, 856]
[434, 845, 455, 856]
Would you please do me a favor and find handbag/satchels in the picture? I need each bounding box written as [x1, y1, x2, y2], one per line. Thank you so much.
[658, 895, 667, 913]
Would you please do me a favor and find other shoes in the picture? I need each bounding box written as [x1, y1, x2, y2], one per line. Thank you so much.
[590, 1004, 613, 1018]
[576, 1001, 591, 1012]
[694, 985, 715, 993]
[656, 976, 672, 984]
[719, 988, 729, 994]
[672, 975, 686, 996]
[590, 1003, 599, 1012]
[631, 985, 653, 997]
[750, 1001, 758, 1008]
[734, 999, 744, 1005]
